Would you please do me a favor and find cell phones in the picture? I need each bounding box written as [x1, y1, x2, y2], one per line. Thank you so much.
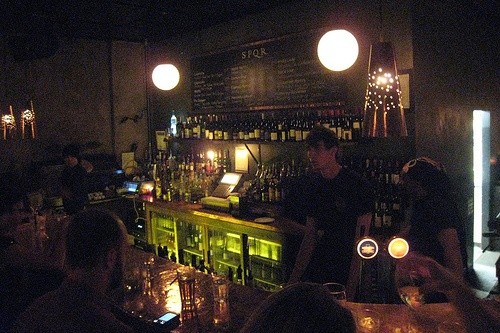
[153, 312, 180, 328]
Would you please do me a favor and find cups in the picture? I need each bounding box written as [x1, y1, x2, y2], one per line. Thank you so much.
[177, 266, 196, 305]
[211, 271, 229, 301]
[323, 282, 440, 333]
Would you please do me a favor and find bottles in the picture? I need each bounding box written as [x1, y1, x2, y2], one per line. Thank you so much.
[133, 225, 148, 240]
[144, 102, 408, 290]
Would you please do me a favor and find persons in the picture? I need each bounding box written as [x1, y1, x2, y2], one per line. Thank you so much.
[274, 126, 373, 300]
[380, 157, 466, 303]
[10, 208, 134, 333]
[63, 143, 96, 213]
[237, 280, 357, 333]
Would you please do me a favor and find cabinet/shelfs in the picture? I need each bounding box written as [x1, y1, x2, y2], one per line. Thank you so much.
[144, 138, 360, 293]
[359, 166, 411, 301]
